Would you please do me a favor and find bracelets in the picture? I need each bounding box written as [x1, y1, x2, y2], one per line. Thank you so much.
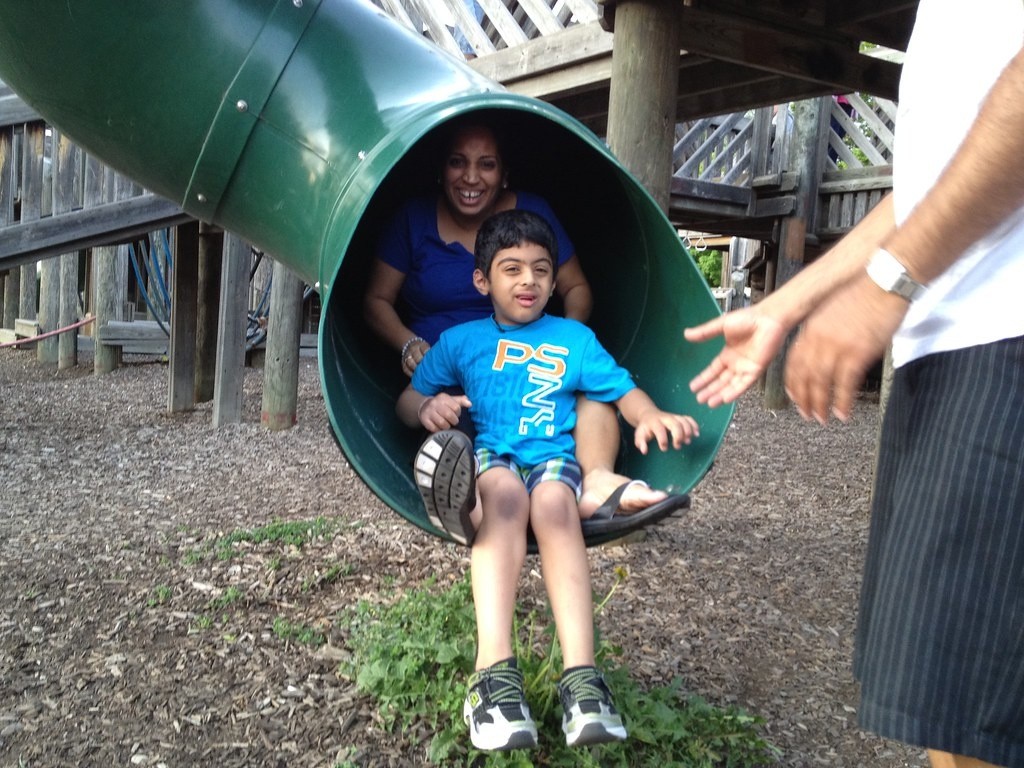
[403, 337, 425, 356]
[417, 396, 434, 417]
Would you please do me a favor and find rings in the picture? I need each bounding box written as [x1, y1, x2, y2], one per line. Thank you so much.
[404, 355, 412, 363]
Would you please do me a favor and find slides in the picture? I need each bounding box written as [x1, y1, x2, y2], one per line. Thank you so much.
[0, 0, 734, 555]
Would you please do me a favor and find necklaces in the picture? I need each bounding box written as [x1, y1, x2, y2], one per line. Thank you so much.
[490, 311, 548, 334]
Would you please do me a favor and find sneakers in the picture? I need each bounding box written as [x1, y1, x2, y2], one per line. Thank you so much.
[557, 668, 627, 746]
[463, 656, 538, 752]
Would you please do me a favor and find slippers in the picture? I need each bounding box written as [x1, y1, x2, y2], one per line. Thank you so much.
[580, 478, 690, 539]
[414, 429, 476, 547]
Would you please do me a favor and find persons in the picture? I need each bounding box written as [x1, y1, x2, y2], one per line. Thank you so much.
[399, 207, 702, 749]
[361, 115, 693, 545]
[681, 0, 1022, 768]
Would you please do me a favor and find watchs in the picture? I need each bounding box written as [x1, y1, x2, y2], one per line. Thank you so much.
[866, 249, 926, 303]
